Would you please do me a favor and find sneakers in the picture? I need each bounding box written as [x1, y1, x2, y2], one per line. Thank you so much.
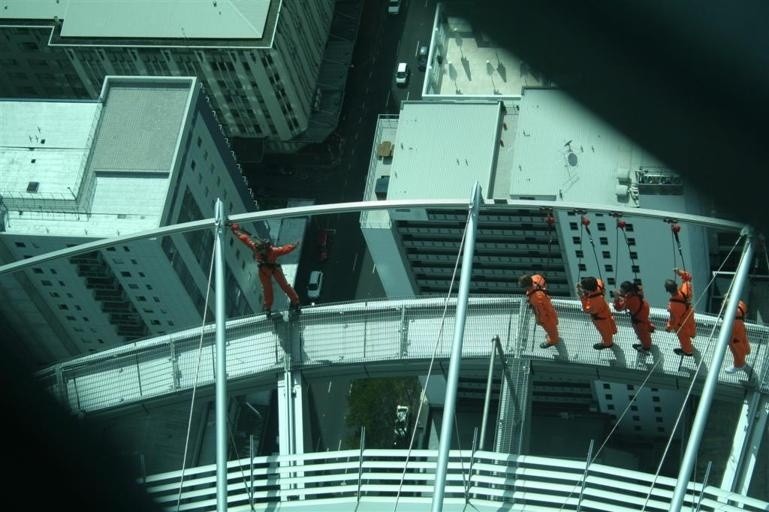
[724, 364, 746, 372]
[632, 343, 650, 352]
[294, 304, 302, 313]
[593, 342, 613, 350]
[539, 342, 553, 348]
[673, 348, 693, 356]
[265, 309, 271, 318]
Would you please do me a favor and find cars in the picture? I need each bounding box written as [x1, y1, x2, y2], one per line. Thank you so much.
[387, 0, 402, 17]
[392, 402, 411, 439]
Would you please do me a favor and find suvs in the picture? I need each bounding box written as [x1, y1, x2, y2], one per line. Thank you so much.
[304, 269, 326, 301]
[416, 45, 430, 72]
[394, 62, 409, 87]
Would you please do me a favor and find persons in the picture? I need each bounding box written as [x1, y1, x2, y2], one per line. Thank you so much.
[518, 275, 561, 348]
[576, 277, 617, 349]
[722, 294, 750, 374]
[228, 223, 302, 319]
[663, 268, 697, 356]
[611, 278, 656, 352]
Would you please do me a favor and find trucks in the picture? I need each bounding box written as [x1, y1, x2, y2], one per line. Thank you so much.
[316, 229, 329, 264]
